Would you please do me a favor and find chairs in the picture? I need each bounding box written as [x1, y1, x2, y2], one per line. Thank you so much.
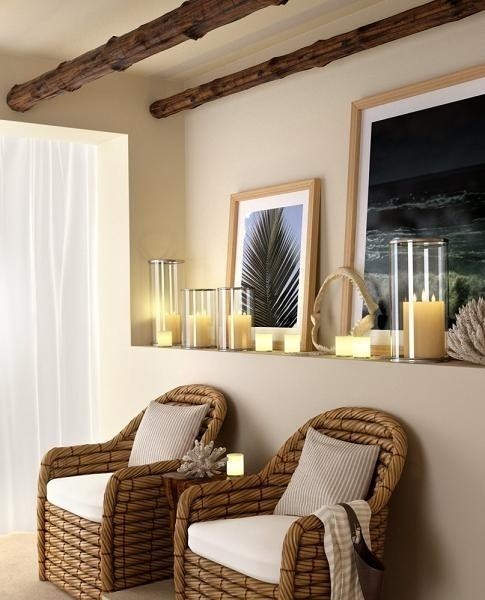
[171, 405, 408, 599]
[37, 380, 227, 600]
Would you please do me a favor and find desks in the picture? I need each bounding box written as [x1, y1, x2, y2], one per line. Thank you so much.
[161, 470, 253, 537]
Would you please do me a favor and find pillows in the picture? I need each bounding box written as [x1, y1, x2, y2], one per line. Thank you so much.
[126, 398, 212, 469]
[268, 424, 384, 520]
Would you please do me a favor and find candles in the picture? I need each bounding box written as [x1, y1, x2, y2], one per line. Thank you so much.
[226, 309, 252, 349]
[402, 291, 446, 359]
[188, 310, 213, 347]
[156, 309, 180, 345]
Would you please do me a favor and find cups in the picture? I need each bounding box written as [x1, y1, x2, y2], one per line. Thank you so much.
[226, 452, 245, 477]
[335, 336, 353, 358]
[255, 334, 272, 351]
[284, 334, 301, 353]
[353, 338, 370, 359]
[157, 330, 172, 347]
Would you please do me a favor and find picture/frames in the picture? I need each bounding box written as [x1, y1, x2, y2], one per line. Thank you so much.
[224, 178, 322, 352]
[339, 61, 485, 357]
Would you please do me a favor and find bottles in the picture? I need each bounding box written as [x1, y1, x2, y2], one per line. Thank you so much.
[215, 287, 255, 351]
[181, 287, 219, 350]
[148, 259, 187, 347]
[391, 235, 449, 364]
